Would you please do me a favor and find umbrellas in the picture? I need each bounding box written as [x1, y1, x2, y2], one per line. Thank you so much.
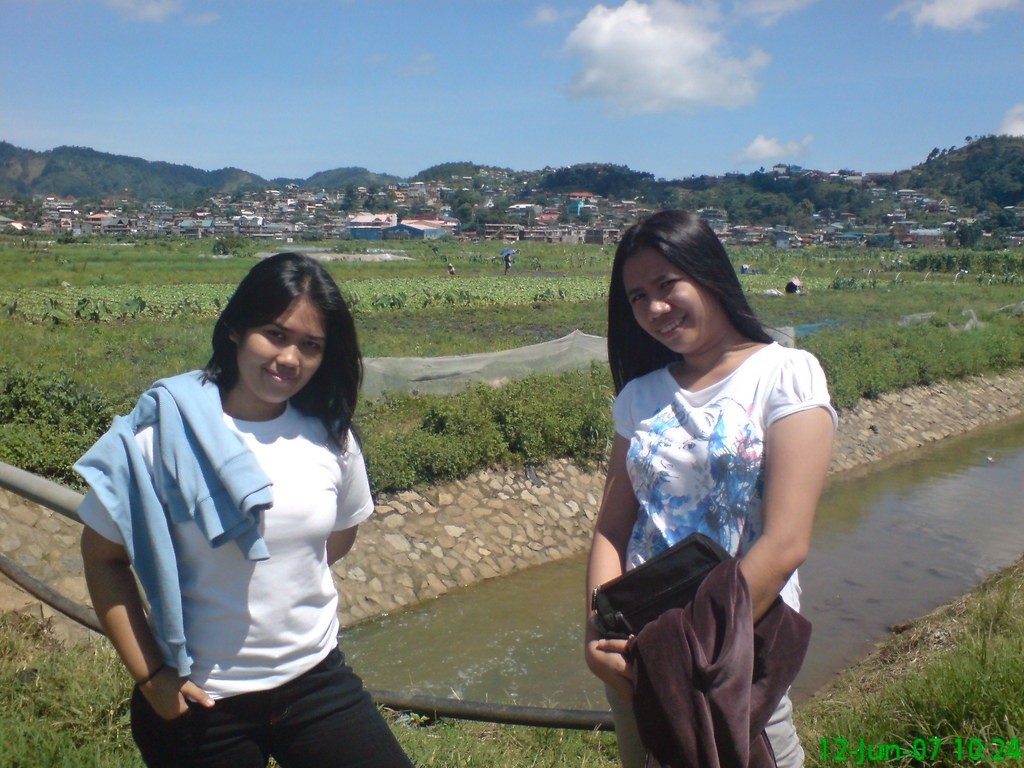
[501, 249, 515, 255]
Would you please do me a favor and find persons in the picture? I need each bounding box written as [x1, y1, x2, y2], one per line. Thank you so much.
[505, 253, 511, 275]
[448, 264, 455, 275]
[785, 279, 801, 293]
[72, 252, 414, 768]
[741, 264, 749, 273]
[586, 209, 839, 768]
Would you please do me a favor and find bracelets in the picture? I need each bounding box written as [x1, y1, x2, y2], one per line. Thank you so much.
[137, 665, 164, 686]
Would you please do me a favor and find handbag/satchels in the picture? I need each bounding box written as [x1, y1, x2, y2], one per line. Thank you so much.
[590, 531, 732, 642]
